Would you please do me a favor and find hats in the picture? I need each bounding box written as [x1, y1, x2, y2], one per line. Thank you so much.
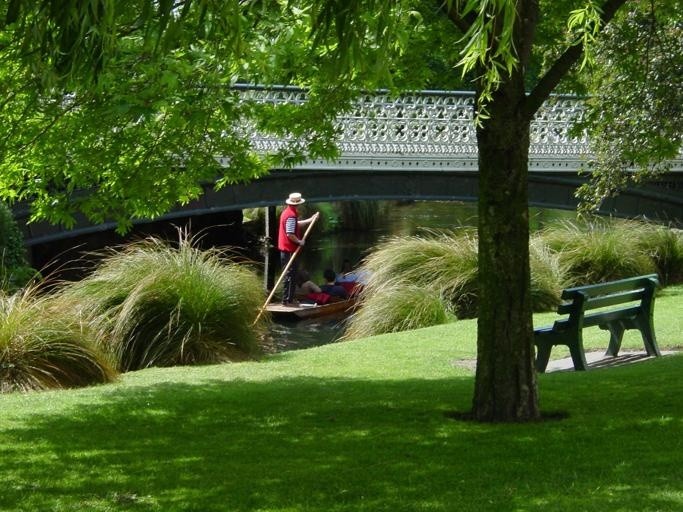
[284, 192, 305, 206]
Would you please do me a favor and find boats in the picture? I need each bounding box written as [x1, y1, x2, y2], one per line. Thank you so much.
[252, 270, 365, 323]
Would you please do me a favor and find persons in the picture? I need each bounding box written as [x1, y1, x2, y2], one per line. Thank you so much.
[334, 259, 356, 283]
[319, 268, 347, 301]
[293, 266, 321, 297]
[277, 193, 319, 304]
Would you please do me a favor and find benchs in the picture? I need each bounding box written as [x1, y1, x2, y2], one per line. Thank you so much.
[533, 273, 664, 373]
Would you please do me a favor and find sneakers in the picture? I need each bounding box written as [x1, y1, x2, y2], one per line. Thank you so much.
[282, 300, 299, 307]
